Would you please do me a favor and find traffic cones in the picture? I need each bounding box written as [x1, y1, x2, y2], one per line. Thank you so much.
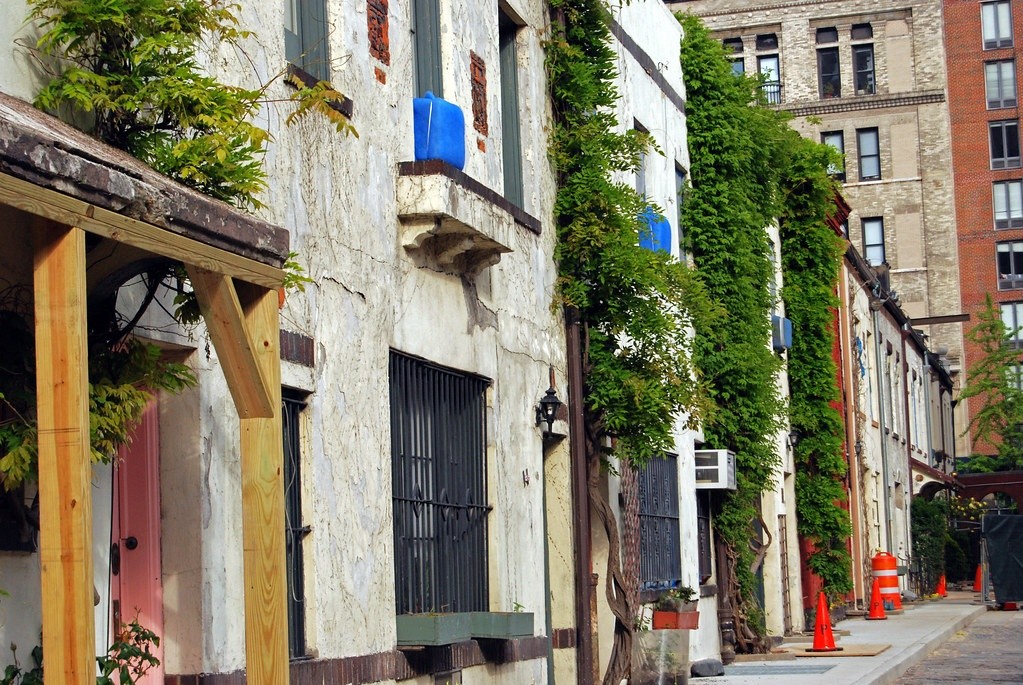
[804, 592, 844, 652]
[864, 577, 888, 620]
[972, 565, 984, 592]
[933, 576, 949, 598]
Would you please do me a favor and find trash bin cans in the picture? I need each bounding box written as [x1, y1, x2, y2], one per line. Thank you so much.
[870, 551, 902, 610]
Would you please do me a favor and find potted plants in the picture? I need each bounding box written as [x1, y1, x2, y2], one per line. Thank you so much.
[656, 586, 700, 612]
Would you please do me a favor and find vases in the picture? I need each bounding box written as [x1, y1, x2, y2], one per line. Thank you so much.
[652, 610, 700, 629]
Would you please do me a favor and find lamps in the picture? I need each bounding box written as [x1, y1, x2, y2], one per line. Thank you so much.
[786, 429, 799, 451]
[535, 385, 562, 441]
[854, 441, 863, 456]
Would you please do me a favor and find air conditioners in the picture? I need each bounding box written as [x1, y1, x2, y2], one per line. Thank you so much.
[695, 448, 737, 490]
